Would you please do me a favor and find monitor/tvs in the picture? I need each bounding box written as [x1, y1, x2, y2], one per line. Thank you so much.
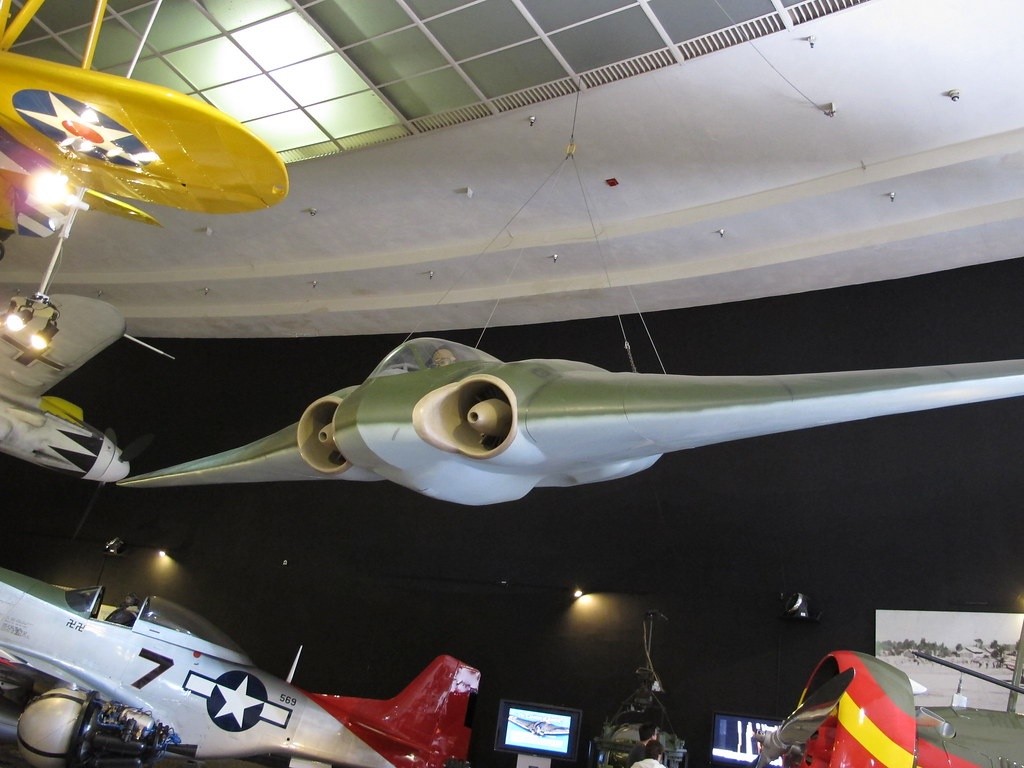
[494, 698, 582, 761]
[709, 710, 788, 768]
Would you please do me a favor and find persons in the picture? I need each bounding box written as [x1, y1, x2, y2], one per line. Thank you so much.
[979, 660, 1003, 668]
[425, 346, 460, 369]
[631, 740, 666, 768]
[624, 724, 657, 768]
[105, 592, 141, 627]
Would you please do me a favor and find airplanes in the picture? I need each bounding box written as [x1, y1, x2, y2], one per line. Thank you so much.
[0, 1, 288, 484]
[114, 337, 1024, 507]
[0, 568, 482, 768]
[751, 650, 985, 768]
